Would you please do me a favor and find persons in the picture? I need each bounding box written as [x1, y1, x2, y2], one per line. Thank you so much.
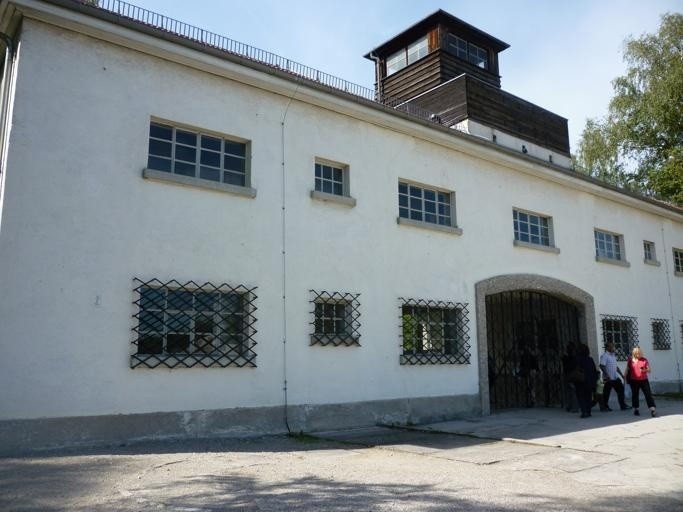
[591, 371, 612, 412]
[561, 342, 578, 414]
[623, 346, 657, 417]
[599, 342, 631, 411]
[574, 344, 597, 418]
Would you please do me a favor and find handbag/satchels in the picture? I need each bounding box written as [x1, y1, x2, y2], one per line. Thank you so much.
[626, 370, 630, 384]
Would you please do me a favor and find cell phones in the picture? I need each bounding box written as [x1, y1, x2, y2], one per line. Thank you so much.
[640, 366, 643, 370]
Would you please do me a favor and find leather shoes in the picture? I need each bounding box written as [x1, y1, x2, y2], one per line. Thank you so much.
[621, 404, 639, 415]
[600, 407, 612, 411]
